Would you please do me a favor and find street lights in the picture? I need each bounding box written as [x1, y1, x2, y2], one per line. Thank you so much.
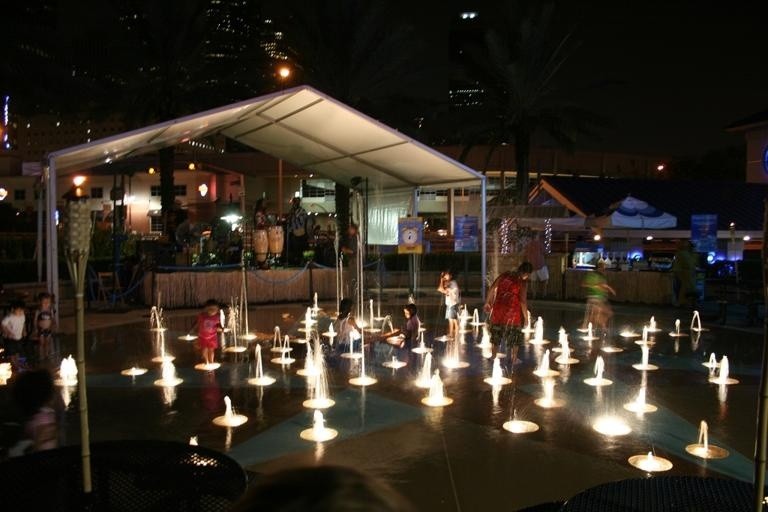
[729, 221, 741, 303]
[274, 59, 294, 219]
[60, 192, 98, 494]
[308, 202, 331, 224]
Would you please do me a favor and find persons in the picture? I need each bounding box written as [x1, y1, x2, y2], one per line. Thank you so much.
[254, 197, 270, 229]
[483, 259, 532, 363]
[581, 258, 616, 333]
[1, 292, 66, 452]
[185, 299, 228, 364]
[438, 270, 461, 339]
[339, 225, 358, 266]
[286, 197, 307, 245]
[400, 305, 420, 367]
[334, 299, 371, 368]
[671, 239, 699, 304]
[523, 230, 551, 299]
[175, 210, 201, 245]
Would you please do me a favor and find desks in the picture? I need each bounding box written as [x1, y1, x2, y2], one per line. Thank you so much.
[1, 440, 247, 512]
[560, 477, 768, 512]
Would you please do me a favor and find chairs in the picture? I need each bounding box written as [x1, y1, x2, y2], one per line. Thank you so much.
[96, 271, 125, 311]
